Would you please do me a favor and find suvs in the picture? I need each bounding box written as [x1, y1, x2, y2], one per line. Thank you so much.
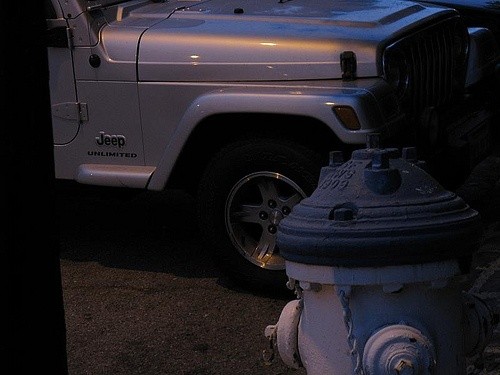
[44, 1, 495, 291]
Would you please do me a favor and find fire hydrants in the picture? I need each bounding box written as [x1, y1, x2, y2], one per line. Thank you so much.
[264, 131, 494, 375]
